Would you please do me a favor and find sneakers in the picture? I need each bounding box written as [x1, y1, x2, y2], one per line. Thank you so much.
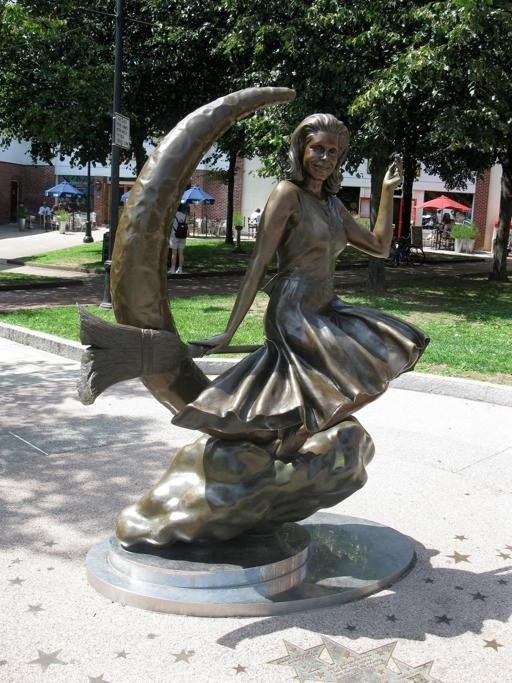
[168, 269, 182, 274]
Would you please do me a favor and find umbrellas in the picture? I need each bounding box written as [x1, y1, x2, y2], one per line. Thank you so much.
[121, 189, 131, 201]
[45, 182, 83, 198]
[181, 185, 214, 204]
[414, 195, 472, 213]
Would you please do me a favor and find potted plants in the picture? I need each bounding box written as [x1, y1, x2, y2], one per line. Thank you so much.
[55, 208, 71, 233]
[16, 206, 30, 230]
[447, 220, 481, 253]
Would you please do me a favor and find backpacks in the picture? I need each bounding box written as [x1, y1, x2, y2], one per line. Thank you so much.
[173, 215, 188, 239]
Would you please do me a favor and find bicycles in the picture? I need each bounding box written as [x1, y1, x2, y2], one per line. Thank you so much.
[384, 227, 425, 269]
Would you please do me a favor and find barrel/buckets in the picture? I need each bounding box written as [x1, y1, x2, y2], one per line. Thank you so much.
[454, 238, 477, 254]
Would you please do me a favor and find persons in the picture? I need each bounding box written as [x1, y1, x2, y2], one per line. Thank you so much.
[437, 210, 454, 239]
[171, 113, 430, 462]
[40, 202, 81, 215]
[249, 208, 261, 227]
[17, 202, 32, 229]
[167, 204, 190, 274]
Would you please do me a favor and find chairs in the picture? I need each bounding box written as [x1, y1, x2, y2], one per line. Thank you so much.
[247, 216, 258, 238]
[75, 209, 97, 231]
